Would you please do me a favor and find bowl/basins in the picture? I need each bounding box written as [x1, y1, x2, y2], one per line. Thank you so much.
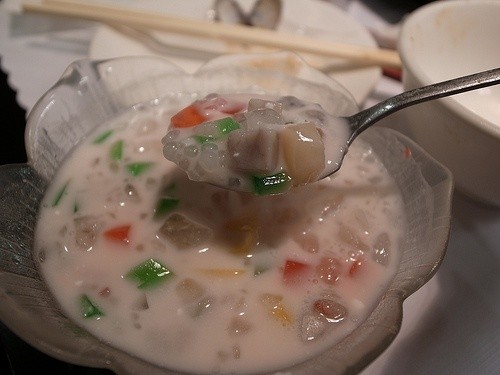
[0, 53, 453, 375]
[397, 1, 500, 209]
[88, 1, 383, 116]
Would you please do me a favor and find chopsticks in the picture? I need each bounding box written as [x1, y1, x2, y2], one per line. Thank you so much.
[21, 0, 405, 68]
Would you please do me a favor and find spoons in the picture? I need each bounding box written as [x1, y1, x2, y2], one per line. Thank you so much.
[163, 69, 500, 193]
